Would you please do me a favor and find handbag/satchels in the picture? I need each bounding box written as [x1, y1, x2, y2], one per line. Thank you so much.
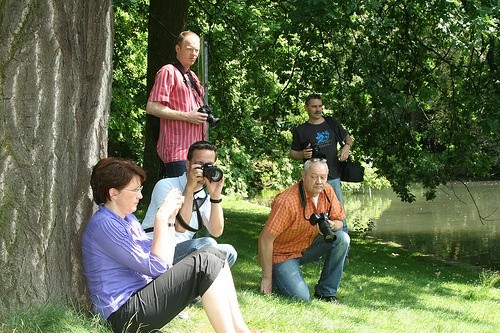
[338, 160, 364, 182]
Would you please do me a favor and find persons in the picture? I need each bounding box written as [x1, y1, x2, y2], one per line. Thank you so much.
[81, 159, 249, 333]
[257, 158, 350, 303]
[289, 94, 355, 267]
[146, 32, 208, 177]
[140, 142, 237, 268]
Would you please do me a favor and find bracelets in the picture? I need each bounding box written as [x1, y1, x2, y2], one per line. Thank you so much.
[345, 143, 352, 148]
[210, 198, 222, 203]
[168, 223, 176, 227]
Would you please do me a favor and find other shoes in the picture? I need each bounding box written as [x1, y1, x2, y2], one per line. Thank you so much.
[314, 292, 338, 302]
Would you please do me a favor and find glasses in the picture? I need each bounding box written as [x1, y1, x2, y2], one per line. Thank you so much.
[309, 158, 327, 163]
[122, 185, 143, 193]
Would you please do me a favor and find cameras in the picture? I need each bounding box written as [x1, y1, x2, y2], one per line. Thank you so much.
[196, 163, 223, 182]
[307, 146, 326, 160]
[309, 212, 337, 243]
[198, 105, 221, 126]
